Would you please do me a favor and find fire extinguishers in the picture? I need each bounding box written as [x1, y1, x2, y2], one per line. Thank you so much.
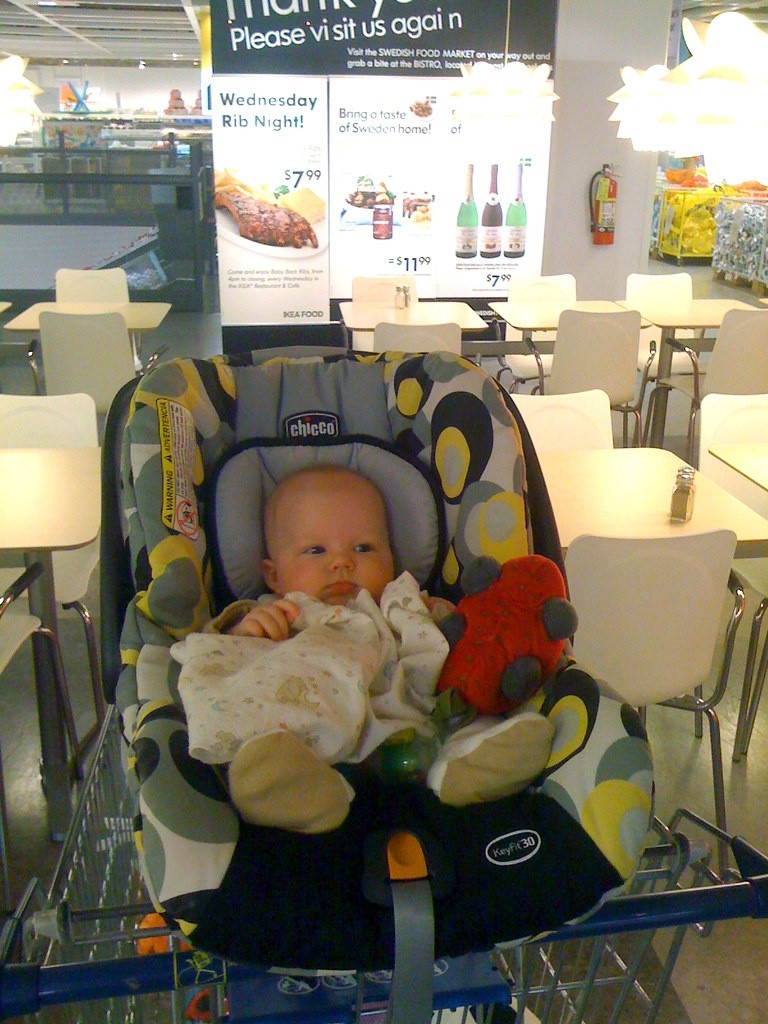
[589, 164, 621, 245]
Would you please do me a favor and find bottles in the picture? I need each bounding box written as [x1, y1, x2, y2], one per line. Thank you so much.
[456, 164, 478, 258]
[504, 164, 527, 258]
[373, 204, 393, 239]
[670, 467, 695, 521]
[480, 165, 502, 258]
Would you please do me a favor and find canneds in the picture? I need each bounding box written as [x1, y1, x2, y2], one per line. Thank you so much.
[372, 205, 393, 239]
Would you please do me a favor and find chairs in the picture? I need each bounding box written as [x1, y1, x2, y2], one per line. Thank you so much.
[0, 269, 768, 961]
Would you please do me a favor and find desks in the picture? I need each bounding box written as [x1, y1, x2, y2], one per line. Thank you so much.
[0, 393, 105, 841]
[338, 300, 488, 353]
[614, 299, 759, 448]
[538, 446, 768, 560]
[4, 301, 173, 395]
[709, 451, 768, 496]
[489, 302, 653, 395]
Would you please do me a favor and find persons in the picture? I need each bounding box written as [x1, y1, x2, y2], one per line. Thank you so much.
[167, 463, 555, 835]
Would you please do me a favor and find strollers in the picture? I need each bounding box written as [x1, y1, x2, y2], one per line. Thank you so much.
[0, 347, 768, 1024]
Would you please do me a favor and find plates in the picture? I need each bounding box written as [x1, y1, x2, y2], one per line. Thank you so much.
[216, 207, 329, 258]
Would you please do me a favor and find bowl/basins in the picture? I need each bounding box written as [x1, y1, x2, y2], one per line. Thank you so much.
[343, 199, 374, 221]
